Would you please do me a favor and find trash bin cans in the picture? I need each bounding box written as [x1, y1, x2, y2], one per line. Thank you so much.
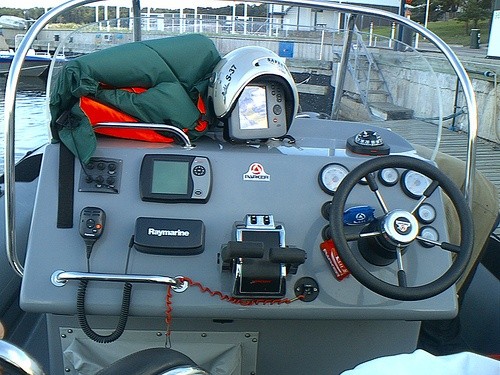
[470, 29, 480, 49]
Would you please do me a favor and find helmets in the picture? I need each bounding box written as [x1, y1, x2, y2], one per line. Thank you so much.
[206, 45, 299, 142]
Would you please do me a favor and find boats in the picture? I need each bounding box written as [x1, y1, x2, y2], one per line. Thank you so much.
[14, 34, 74, 79]
[0, 34, 54, 78]
